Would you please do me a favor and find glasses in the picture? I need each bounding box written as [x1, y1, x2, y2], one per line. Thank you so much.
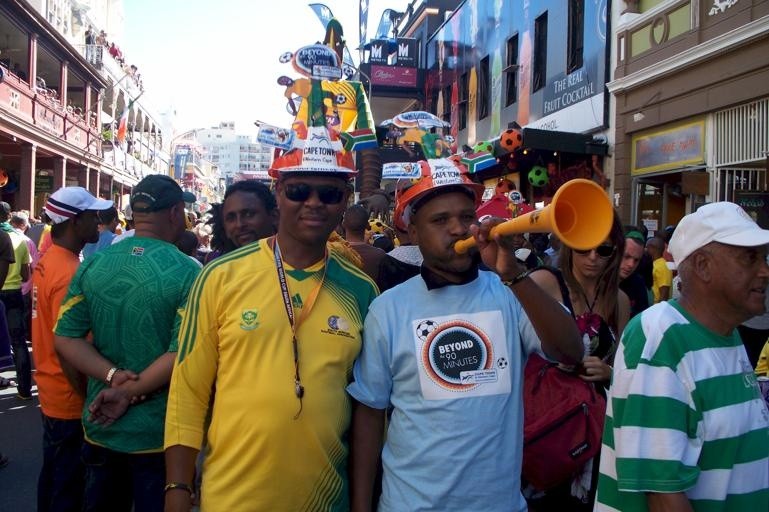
[574, 244, 616, 259]
[280, 183, 344, 204]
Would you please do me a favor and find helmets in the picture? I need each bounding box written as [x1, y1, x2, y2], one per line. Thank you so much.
[393, 157, 485, 233]
[269, 126, 360, 177]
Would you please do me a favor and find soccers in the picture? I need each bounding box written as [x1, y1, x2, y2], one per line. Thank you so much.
[0, 168, 9, 188]
[365, 220, 385, 234]
[445, 135, 454, 143]
[344, 67, 353, 76]
[496, 180, 515, 195]
[416, 320, 438, 342]
[280, 52, 293, 63]
[497, 358, 507, 368]
[528, 165, 549, 188]
[474, 141, 495, 156]
[501, 129, 523, 152]
[336, 93, 346, 104]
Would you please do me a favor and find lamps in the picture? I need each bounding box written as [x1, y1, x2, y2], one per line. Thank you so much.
[633, 112, 644, 122]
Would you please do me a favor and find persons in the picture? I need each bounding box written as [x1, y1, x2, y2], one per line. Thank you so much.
[33, 75, 98, 128]
[0, 47, 12, 76]
[1, 176, 680, 402]
[52, 173, 206, 510]
[162, 130, 380, 511]
[345, 159, 587, 512]
[31, 187, 114, 510]
[277, 77, 338, 114]
[14, 62, 26, 81]
[85, 24, 154, 92]
[522, 209, 632, 512]
[593, 202, 769, 512]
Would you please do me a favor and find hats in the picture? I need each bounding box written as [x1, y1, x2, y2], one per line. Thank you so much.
[125, 205, 133, 220]
[667, 202, 769, 269]
[43, 186, 113, 226]
[513, 248, 537, 267]
[130, 174, 196, 212]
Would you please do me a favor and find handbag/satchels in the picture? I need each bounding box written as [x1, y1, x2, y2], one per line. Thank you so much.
[522, 353, 606, 491]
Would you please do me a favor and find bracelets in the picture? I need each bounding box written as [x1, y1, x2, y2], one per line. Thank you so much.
[162, 482, 196, 497]
